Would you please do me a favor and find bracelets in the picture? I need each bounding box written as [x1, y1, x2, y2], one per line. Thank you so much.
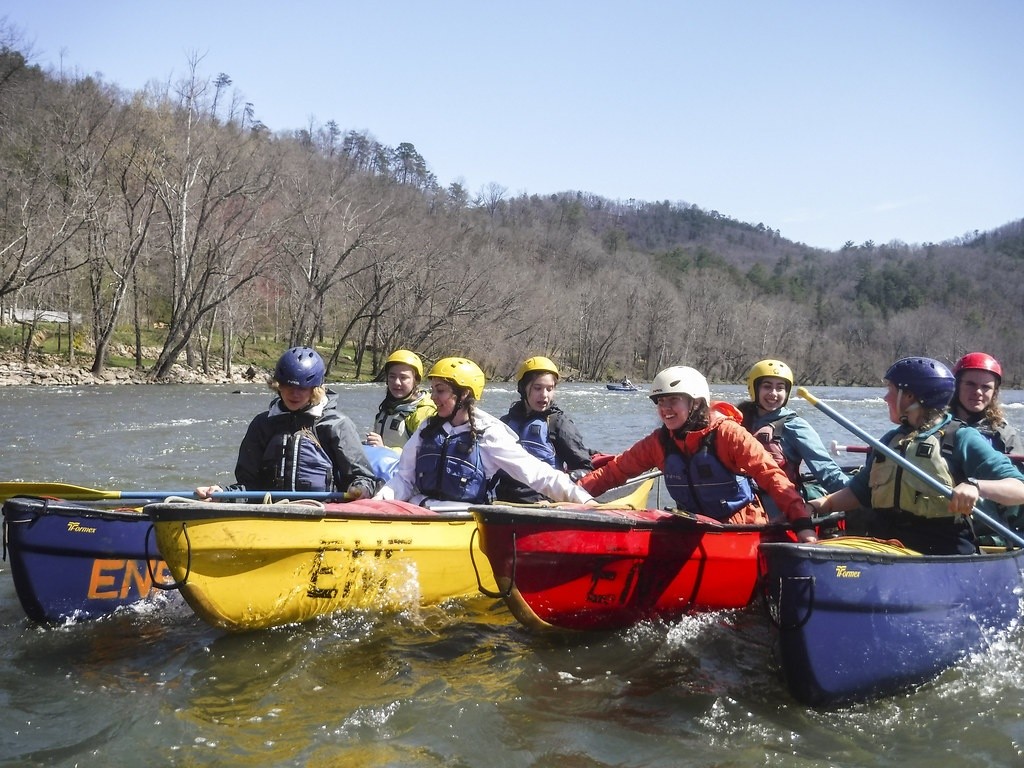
[808, 503, 817, 511]
[825, 496, 833, 512]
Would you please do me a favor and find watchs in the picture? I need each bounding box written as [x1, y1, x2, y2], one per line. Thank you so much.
[965, 477, 979, 489]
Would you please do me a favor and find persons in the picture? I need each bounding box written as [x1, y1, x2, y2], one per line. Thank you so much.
[490, 356, 601, 503]
[737, 359, 850, 517]
[372, 357, 598, 515]
[808, 356, 1024, 555]
[575, 365, 819, 543]
[195, 346, 376, 504]
[365, 350, 438, 454]
[621, 375, 631, 386]
[949, 352, 1024, 455]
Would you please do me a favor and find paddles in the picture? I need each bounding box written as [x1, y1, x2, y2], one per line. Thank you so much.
[795, 383, 1024, 548]
[0, 481, 365, 502]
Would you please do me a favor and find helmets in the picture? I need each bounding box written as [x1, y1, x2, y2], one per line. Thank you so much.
[516, 356, 559, 393]
[273, 346, 325, 387]
[385, 349, 423, 385]
[952, 352, 1001, 417]
[649, 366, 712, 424]
[748, 359, 793, 411]
[884, 357, 956, 426]
[428, 356, 485, 403]
